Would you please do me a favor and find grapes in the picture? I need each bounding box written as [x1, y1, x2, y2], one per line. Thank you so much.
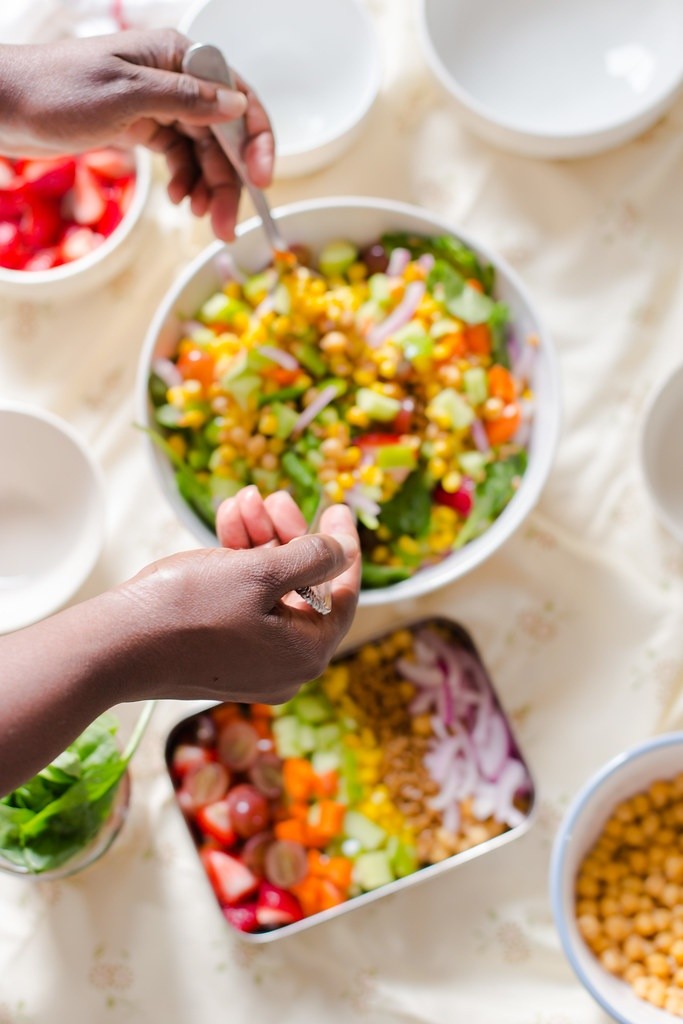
[188, 719, 308, 888]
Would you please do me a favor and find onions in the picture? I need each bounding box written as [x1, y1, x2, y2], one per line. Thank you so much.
[397, 633, 533, 843]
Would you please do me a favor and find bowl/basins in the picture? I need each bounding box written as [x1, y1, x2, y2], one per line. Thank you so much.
[0, 725, 131, 881]
[549, 731, 683, 1022]
[1, 145, 151, 302]
[2, 398, 117, 639]
[175, 1, 387, 183]
[132, 195, 561, 608]
[414, 1, 681, 161]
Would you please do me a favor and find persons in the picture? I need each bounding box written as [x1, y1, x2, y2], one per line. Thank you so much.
[1, 28, 362, 798]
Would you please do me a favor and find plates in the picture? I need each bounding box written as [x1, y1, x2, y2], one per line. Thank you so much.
[163, 615, 543, 945]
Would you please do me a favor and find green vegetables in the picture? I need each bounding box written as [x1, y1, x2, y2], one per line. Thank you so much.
[0, 700, 153, 875]
[146, 223, 529, 590]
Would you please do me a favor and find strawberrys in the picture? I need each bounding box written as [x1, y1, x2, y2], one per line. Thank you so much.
[170, 745, 304, 931]
[0, 148, 133, 273]
